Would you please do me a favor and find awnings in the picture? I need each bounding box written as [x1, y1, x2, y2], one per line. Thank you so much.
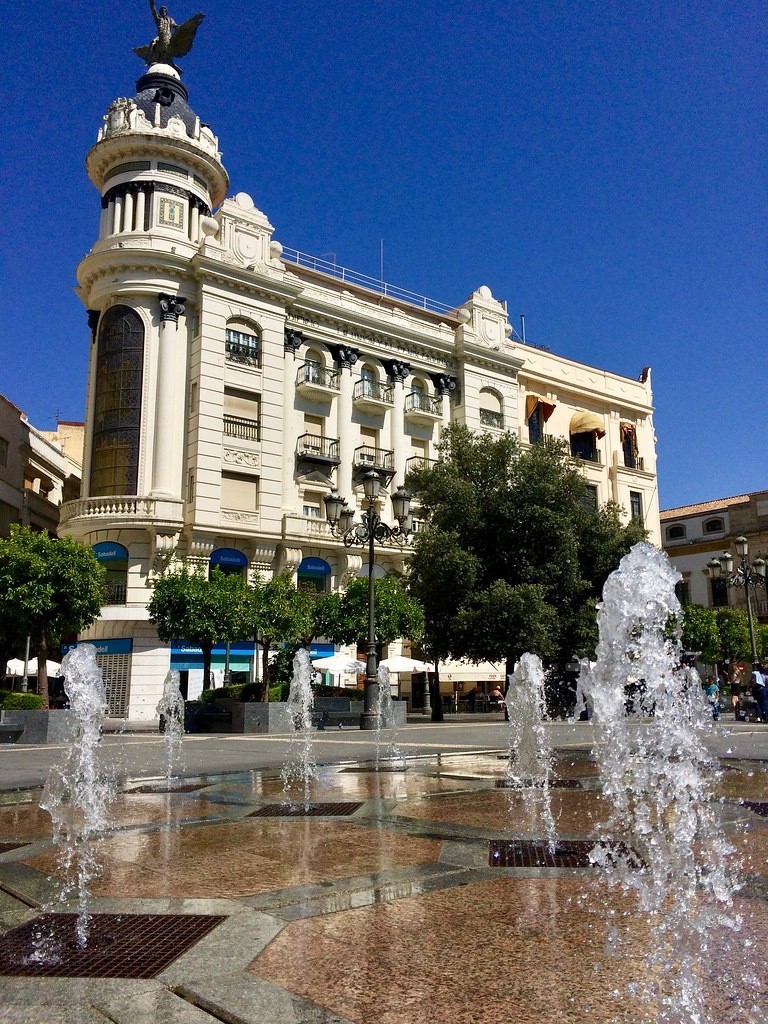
[438, 656, 518, 682]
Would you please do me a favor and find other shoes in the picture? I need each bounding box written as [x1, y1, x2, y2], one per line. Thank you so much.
[755, 717, 766, 723]
[714, 718, 718, 721]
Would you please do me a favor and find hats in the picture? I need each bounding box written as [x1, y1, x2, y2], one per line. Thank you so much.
[496, 686, 501, 689]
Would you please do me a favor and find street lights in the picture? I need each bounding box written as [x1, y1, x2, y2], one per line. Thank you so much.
[706, 534, 767, 671]
[323, 465, 412, 731]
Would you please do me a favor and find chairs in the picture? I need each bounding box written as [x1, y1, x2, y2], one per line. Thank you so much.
[439, 695, 506, 713]
[402, 696, 409, 714]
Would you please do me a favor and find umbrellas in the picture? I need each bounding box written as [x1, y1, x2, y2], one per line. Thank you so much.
[379, 657, 435, 686]
[312, 652, 367, 687]
[6, 657, 63, 694]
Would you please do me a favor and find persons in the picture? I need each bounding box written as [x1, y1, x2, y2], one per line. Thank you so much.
[704, 663, 768, 723]
[468, 685, 503, 714]
[144, 0, 180, 66]
